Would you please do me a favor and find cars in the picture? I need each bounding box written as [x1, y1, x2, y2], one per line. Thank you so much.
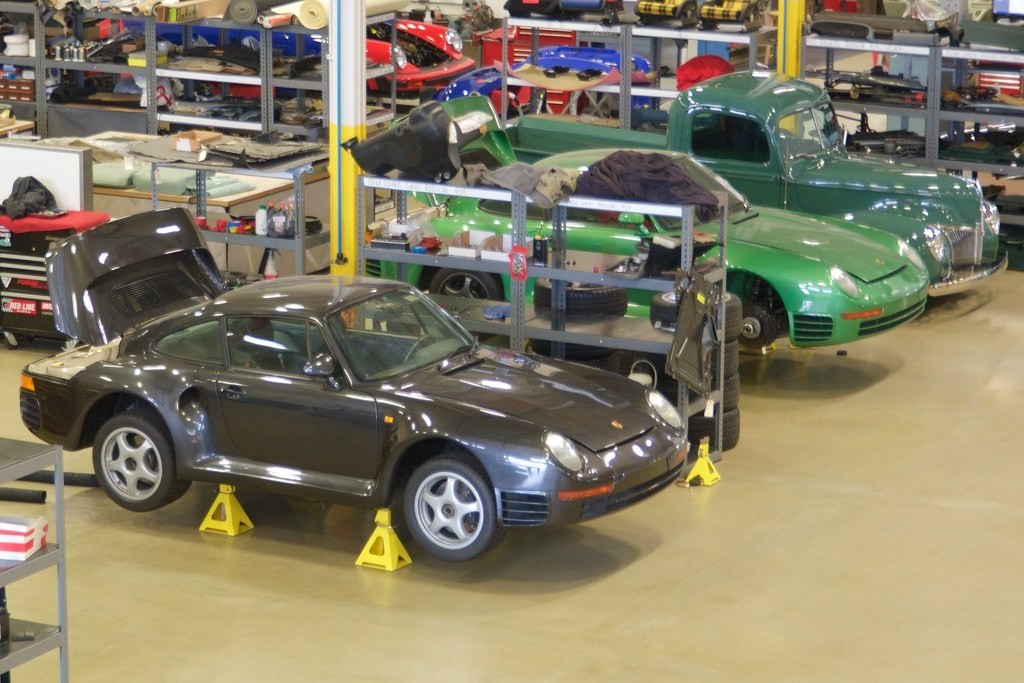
[366, 93, 932, 353]
[434, 44, 653, 121]
[19, 206, 692, 565]
[365, 17, 477, 98]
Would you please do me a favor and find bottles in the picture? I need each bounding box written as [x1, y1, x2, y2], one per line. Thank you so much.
[264, 251, 278, 278]
[256, 199, 295, 238]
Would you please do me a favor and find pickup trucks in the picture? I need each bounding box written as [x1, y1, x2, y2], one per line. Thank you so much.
[507, 65, 1009, 298]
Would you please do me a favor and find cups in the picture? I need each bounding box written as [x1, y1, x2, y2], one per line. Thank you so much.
[196, 216, 205, 228]
[216, 219, 245, 234]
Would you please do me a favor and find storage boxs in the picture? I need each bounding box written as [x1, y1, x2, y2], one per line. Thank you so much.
[0, 514, 50, 563]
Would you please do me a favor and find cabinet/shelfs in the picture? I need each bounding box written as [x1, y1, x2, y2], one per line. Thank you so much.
[0, 0, 1024, 482]
[0, 437, 70, 683]
